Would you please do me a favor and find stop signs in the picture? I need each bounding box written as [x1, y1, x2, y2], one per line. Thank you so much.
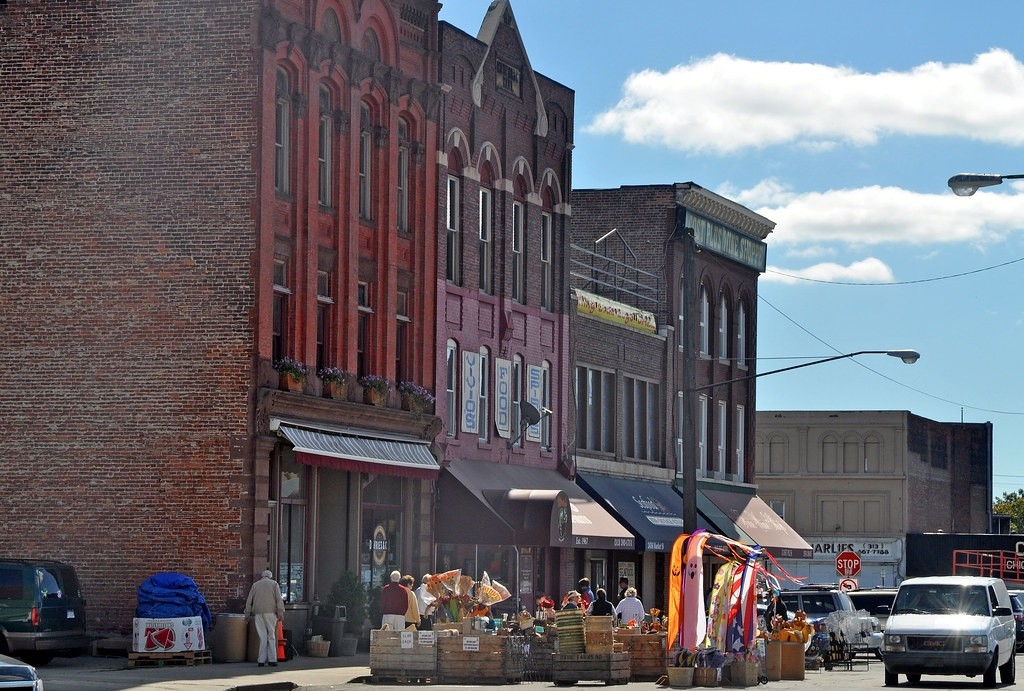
[836, 552, 862, 578]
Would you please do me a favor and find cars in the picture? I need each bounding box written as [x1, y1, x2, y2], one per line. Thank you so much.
[1007, 590, 1024, 653]
[0, 654, 43, 691]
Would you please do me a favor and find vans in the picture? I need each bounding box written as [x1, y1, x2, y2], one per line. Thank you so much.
[0, 557, 87, 666]
[876, 576, 1017, 688]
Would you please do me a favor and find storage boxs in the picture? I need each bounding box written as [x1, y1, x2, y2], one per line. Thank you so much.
[131, 616, 206, 653]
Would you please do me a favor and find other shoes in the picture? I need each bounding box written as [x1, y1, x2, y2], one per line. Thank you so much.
[268, 662, 279, 666]
[258, 663, 265, 666]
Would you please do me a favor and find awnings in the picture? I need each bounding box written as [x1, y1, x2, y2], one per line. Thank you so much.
[277, 419, 440, 480]
[698, 489, 814, 557]
[435, 454, 636, 551]
[675, 485, 757, 558]
[579, 470, 730, 554]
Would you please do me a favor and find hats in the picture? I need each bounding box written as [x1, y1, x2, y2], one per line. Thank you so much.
[568, 590, 582, 597]
[617, 576, 629, 585]
[390, 571, 401, 579]
[261, 571, 273, 579]
[420, 574, 432, 583]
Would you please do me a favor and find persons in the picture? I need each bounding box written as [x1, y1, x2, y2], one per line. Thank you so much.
[245, 570, 285, 667]
[379, 570, 437, 631]
[564, 576, 645, 625]
[763, 590, 788, 632]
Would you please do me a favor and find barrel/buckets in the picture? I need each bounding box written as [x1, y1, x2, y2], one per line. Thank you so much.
[214, 613, 246, 662]
[782, 642, 806, 681]
[246, 616, 278, 663]
[693, 667, 719, 687]
[308, 639, 330, 657]
[667, 666, 694, 687]
[766, 641, 782, 681]
[342, 637, 358, 656]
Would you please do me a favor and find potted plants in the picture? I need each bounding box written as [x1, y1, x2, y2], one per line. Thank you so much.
[332, 568, 367, 655]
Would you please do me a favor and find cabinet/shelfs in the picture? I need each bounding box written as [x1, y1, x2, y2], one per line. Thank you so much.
[829, 641, 869, 670]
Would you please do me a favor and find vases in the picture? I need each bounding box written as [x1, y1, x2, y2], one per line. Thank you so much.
[323, 381, 345, 400]
[367, 386, 386, 406]
[278, 373, 303, 393]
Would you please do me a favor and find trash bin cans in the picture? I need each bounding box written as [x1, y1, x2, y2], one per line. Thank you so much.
[283, 603, 313, 650]
[311, 603, 347, 657]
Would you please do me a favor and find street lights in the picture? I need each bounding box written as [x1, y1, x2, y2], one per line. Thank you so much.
[682, 350, 920, 552]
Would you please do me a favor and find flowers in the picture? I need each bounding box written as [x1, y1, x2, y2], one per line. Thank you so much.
[398, 383, 435, 407]
[318, 366, 348, 388]
[357, 376, 391, 397]
[273, 357, 311, 384]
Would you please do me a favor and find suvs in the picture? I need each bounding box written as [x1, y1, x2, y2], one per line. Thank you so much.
[756, 584, 884, 661]
